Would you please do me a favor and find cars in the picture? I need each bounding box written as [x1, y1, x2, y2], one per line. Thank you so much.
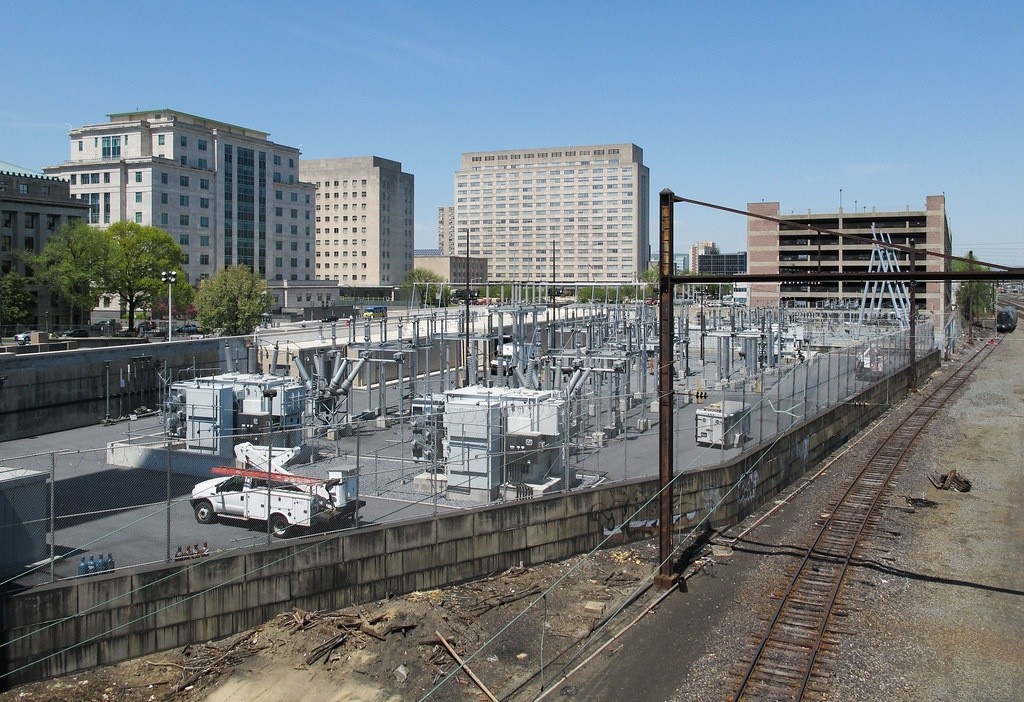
[321, 315, 338, 323]
[177, 324, 197, 335]
[137, 321, 157, 332]
[13, 329, 42, 342]
[90, 319, 122, 331]
[59, 329, 90, 337]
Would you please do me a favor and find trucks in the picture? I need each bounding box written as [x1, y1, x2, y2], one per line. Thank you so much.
[360, 306, 388, 321]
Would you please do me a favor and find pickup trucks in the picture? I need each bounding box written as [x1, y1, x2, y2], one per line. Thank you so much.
[189, 441, 366, 540]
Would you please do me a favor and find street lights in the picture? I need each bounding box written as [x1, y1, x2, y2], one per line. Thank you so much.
[470, 276, 483, 332]
[338, 283, 356, 343]
[161, 270, 177, 341]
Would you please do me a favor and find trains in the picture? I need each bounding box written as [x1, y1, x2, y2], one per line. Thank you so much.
[994, 306, 1018, 333]
